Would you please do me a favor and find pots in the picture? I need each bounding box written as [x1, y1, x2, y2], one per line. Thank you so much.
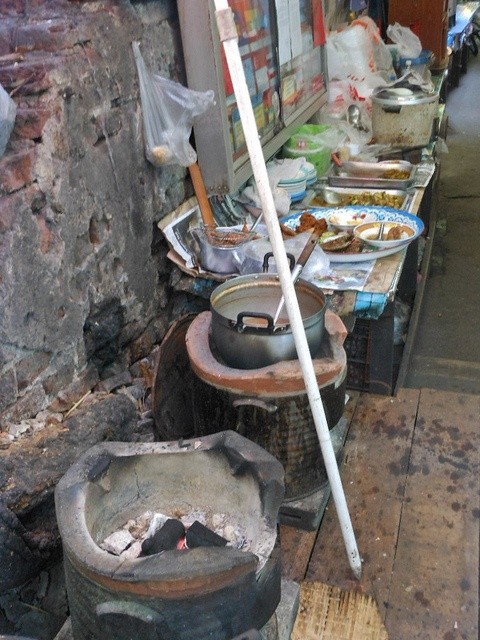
[192, 227, 266, 273]
[210, 252, 327, 369]
[369, 82, 440, 148]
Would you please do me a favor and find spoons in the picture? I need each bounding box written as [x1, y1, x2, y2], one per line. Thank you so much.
[334, 104, 361, 153]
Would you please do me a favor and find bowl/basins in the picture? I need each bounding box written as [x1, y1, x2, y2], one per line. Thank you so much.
[327, 212, 377, 231]
[234, 224, 268, 238]
[353, 221, 417, 249]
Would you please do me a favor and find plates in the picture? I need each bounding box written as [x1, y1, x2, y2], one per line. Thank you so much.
[277, 205, 425, 263]
[267, 158, 317, 187]
[277, 169, 307, 202]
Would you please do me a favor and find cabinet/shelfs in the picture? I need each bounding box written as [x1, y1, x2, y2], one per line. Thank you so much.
[388, 0, 449, 71]
[167, 113, 450, 396]
